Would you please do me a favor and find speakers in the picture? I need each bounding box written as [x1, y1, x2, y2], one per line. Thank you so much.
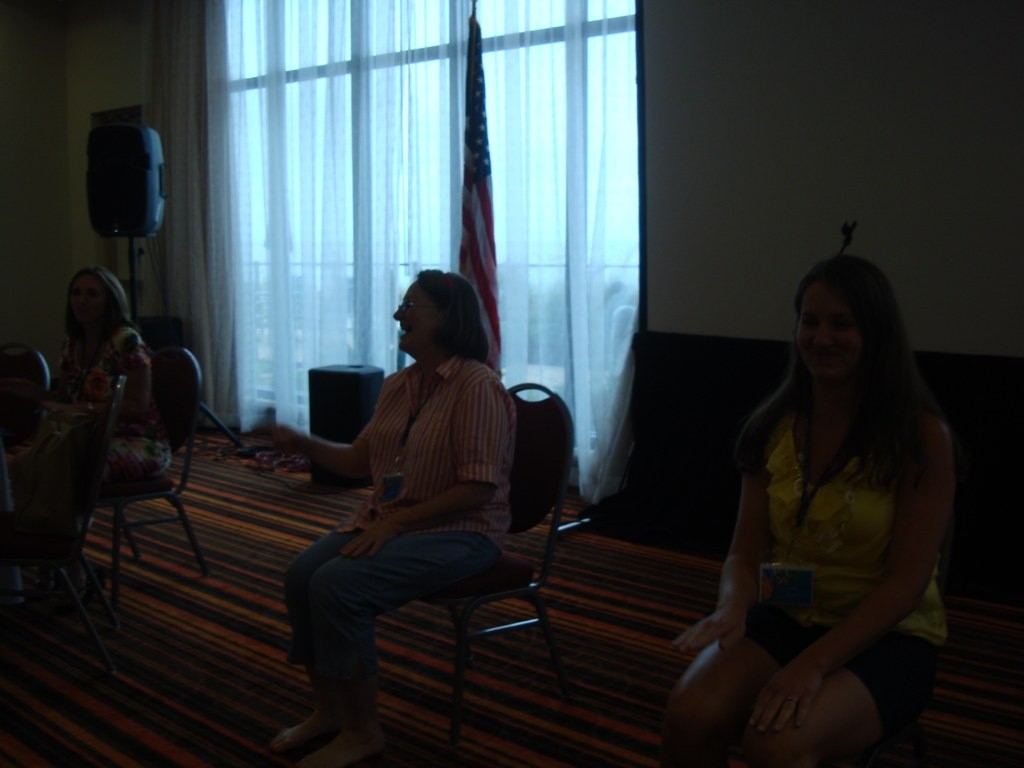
[308, 364, 384, 488]
[87, 122, 166, 238]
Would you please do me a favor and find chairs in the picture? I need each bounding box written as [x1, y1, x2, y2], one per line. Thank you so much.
[0, 341, 51, 443]
[435, 383, 574, 745]
[0, 373, 128, 674]
[95, 344, 210, 610]
[823, 507, 956, 768]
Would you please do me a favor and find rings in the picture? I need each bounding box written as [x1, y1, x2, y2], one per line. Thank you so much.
[785, 695, 798, 705]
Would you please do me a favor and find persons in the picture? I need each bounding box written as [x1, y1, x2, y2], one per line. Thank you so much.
[255, 270, 517, 768]
[661, 255, 956, 768]
[7, 266, 172, 609]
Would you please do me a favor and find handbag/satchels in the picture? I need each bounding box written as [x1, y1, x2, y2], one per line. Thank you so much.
[14, 409, 97, 538]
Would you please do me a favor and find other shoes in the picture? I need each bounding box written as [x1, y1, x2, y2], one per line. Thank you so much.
[62, 560, 88, 611]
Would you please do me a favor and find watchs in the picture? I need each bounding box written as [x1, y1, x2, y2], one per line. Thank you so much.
[88, 402, 94, 413]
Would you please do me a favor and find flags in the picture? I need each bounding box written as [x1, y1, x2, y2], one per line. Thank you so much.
[459, 18, 501, 381]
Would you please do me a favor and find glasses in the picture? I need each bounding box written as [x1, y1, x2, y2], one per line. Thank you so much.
[398, 298, 440, 312]
